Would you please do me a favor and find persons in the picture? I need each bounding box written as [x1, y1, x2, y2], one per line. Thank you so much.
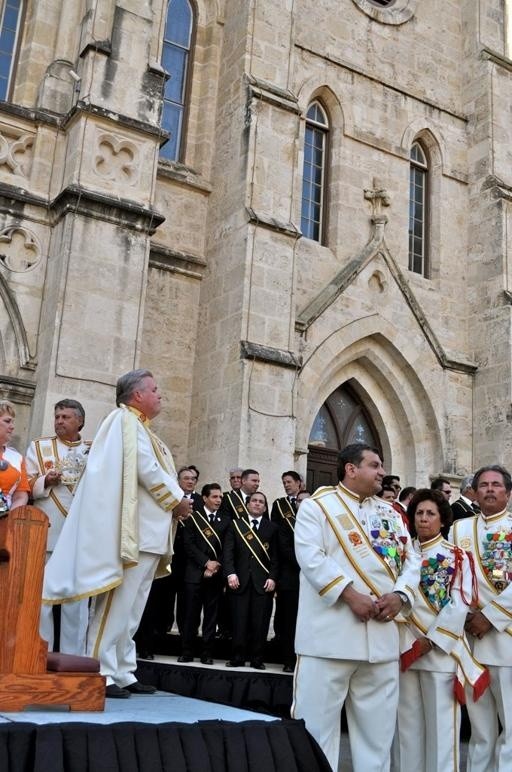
[289, 444, 426, 772]
[134, 457, 482, 670]
[1, 402, 31, 517]
[47, 367, 198, 700]
[449, 464, 510, 772]
[27, 401, 91, 658]
[391, 486, 472, 772]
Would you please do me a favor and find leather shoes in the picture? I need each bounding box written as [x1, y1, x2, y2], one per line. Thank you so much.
[105, 682, 155, 698]
[138, 645, 297, 672]
